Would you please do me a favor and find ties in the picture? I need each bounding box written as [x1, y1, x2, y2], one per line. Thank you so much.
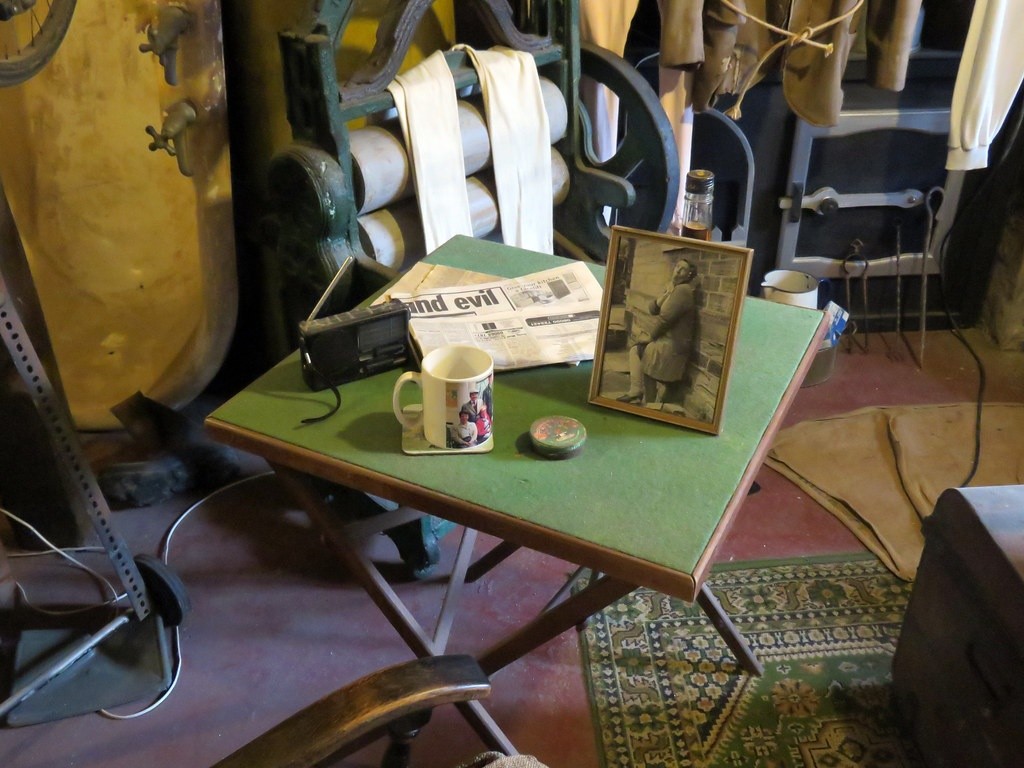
[473, 401, 477, 411]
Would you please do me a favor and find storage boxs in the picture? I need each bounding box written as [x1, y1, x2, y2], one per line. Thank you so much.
[748, 58, 965, 324]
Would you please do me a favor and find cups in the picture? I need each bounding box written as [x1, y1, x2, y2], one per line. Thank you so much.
[391, 344, 494, 450]
[759, 269, 832, 311]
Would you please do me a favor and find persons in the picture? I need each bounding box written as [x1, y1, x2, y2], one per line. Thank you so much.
[615, 256, 698, 407]
[461, 388, 484, 423]
[475, 405, 492, 442]
[451, 410, 478, 448]
[481, 374, 493, 421]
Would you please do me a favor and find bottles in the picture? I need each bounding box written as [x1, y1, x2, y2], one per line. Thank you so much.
[681, 169, 715, 242]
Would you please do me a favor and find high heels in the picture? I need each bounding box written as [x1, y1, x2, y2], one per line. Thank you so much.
[616, 391, 644, 403]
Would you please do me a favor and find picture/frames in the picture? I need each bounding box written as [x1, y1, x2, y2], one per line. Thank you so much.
[588, 225, 755, 436]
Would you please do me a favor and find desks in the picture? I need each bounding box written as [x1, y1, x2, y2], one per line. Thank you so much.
[198, 228, 833, 768]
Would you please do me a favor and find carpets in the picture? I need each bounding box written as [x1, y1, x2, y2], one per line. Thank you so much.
[564, 546, 967, 768]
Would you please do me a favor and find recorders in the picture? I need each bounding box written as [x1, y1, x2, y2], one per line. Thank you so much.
[298, 257, 411, 391]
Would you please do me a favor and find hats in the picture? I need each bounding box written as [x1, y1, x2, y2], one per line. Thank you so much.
[470, 388, 478, 393]
